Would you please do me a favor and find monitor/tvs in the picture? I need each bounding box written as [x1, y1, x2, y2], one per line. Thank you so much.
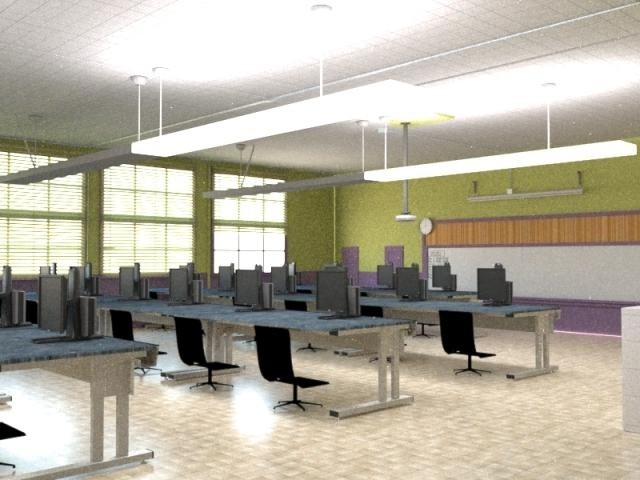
[0, 263, 512, 345]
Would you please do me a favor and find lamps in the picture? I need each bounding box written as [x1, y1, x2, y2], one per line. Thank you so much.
[0, 5, 640, 203]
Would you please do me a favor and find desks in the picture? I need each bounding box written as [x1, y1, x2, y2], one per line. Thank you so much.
[0, 321, 155, 480]
[107, 294, 414, 419]
[151, 286, 561, 380]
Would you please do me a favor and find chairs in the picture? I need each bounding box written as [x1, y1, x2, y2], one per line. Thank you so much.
[284, 298, 327, 351]
[360, 305, 401, 363]
[169, 314, 240, 390]
[437, 308, 498, 376]
[108, 309, 162, 375]
[253, 324, 331, 413]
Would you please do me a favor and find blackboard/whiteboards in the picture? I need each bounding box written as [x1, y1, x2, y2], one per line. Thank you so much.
[426, 242, 640, 305]
